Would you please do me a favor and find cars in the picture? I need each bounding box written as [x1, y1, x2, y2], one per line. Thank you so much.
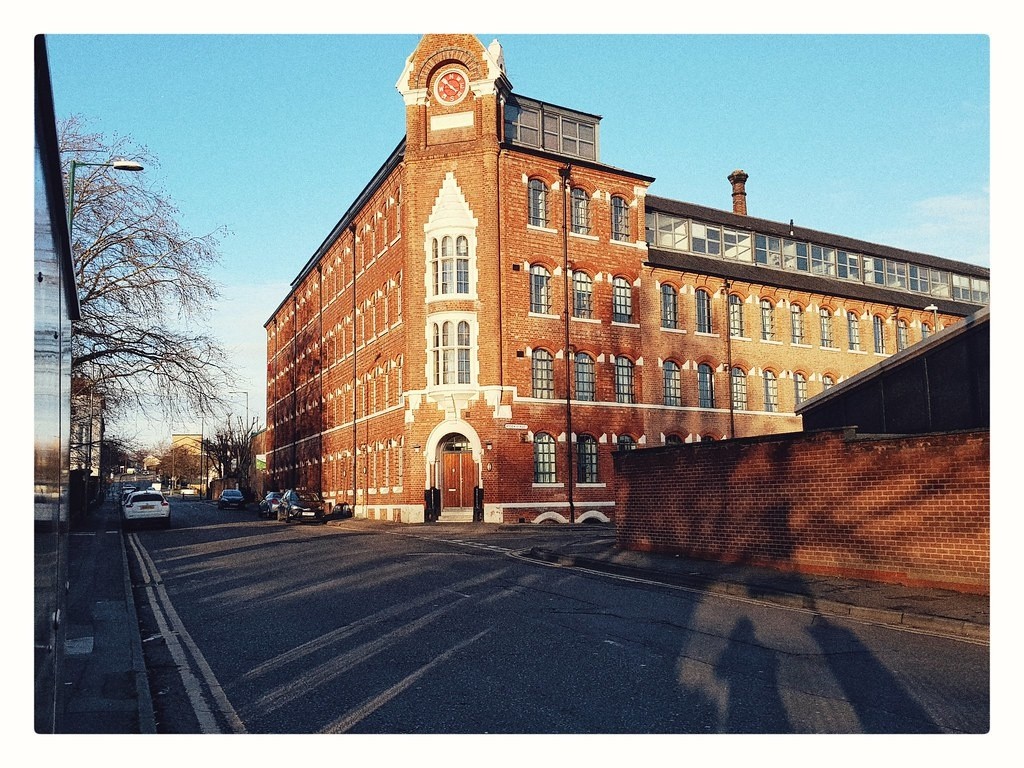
[258, 492, 284, 519]
[119, 484, 171, 526]
[277, 489, 325, 523]
[217, 489, 246, 511]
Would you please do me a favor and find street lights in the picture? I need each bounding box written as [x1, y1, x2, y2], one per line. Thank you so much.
[228, 392, 248, 488]
[197, 417, 204, 500]
[83, 341, 125, 525]
[68, 160, 143, 246]
